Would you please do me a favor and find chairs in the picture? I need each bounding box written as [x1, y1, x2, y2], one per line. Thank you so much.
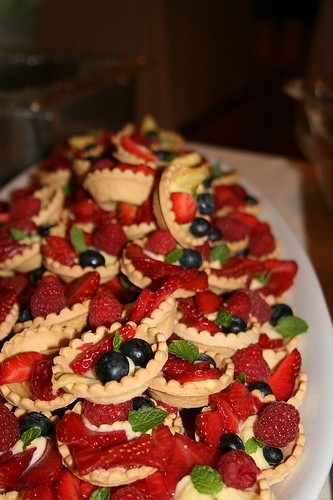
[30, 66, 138, 164]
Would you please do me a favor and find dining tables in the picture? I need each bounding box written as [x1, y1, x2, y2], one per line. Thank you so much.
[194, 154, 333, 331]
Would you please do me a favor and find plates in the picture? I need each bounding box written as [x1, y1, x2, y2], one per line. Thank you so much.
[2, 150, 332, 500]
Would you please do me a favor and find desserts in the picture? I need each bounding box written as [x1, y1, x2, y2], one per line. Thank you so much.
[0, 115, 310, 500]
